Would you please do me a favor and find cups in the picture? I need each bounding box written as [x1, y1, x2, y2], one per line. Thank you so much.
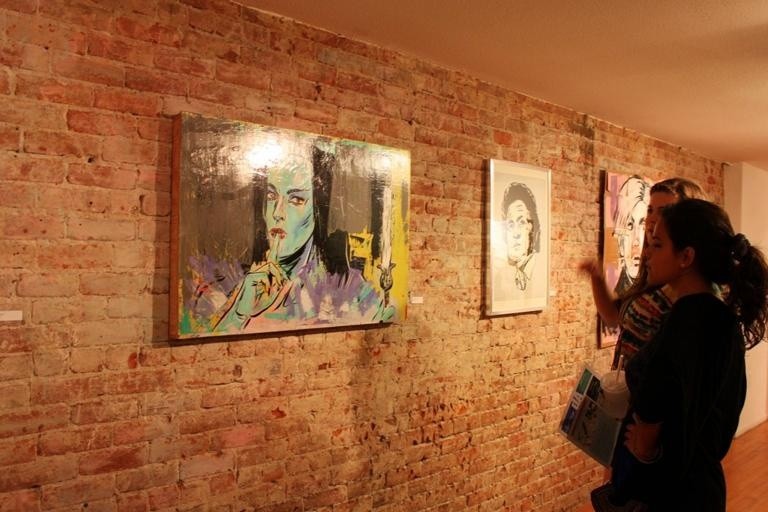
[600, 370, 629, 420]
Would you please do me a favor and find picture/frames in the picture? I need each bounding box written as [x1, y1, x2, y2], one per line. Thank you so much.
[486, 157, 553, 317]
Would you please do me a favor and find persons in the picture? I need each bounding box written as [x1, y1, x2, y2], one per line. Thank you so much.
[576, 176, 712, 371]
[188, 126, 398, 338]
[499, 179, 542, 293]
[590, 197, 768, 512]
[606, 173, 654, 331]
